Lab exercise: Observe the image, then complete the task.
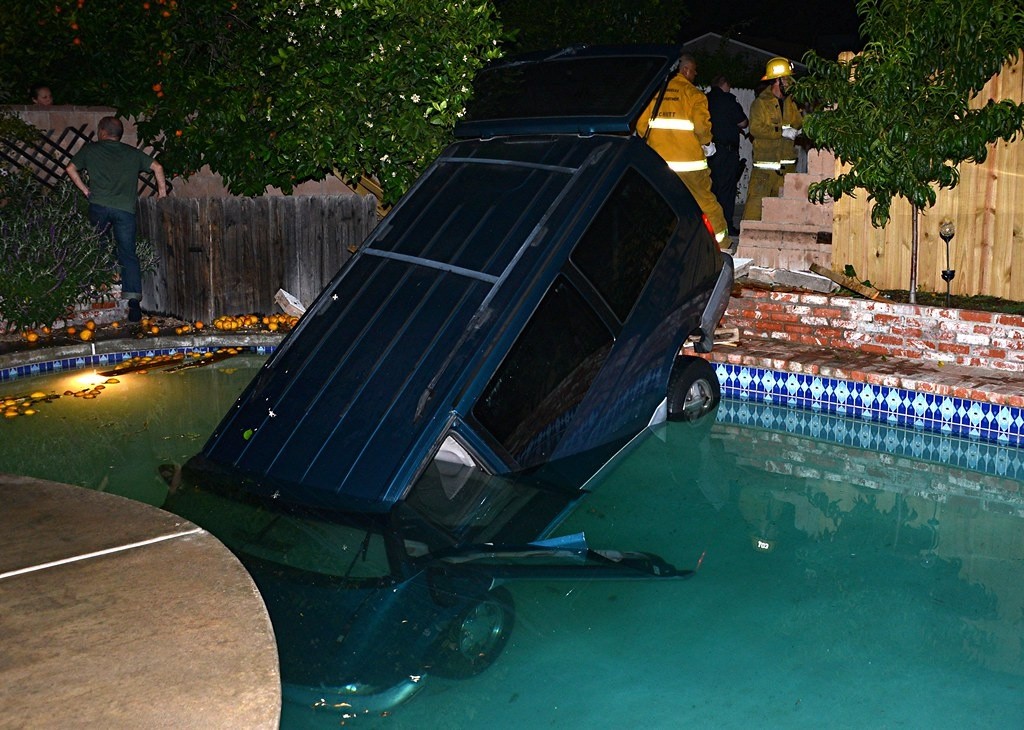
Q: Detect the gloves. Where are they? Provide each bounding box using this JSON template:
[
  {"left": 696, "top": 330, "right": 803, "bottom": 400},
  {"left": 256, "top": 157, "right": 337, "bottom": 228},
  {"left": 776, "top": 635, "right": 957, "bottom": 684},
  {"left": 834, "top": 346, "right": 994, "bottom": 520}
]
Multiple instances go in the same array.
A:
[
  {"left": 781, "top": 127, "right": 798, "bottom": 140},
  {"left": 701, "top": 142, "right": 716, "bottom": 157}
]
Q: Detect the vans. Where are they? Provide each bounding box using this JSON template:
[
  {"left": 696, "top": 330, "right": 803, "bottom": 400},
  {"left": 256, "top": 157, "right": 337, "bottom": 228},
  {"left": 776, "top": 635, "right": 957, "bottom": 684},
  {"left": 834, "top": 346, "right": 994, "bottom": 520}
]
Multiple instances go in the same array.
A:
[{"left": 157, "top": 37, "right": 736, "bottom": 717}]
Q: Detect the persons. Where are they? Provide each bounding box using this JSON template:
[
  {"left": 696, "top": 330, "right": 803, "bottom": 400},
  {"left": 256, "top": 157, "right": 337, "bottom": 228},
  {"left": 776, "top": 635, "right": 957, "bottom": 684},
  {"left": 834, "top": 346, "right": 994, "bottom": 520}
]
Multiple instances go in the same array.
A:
[
  {"left": 705, "top": 72, "right": 748, "bottom": 237},
  {"left": 741, "top": 56, "right": 803, "bottom": 219},
  {"left": 66, "top": 116, "right": 168, "bottom": 322},
  {"left": 636, "top": 56, "right": 732, "bottom": 248},
  {"left": 30, "top": 85, "right": 53, "bottom": 105}
]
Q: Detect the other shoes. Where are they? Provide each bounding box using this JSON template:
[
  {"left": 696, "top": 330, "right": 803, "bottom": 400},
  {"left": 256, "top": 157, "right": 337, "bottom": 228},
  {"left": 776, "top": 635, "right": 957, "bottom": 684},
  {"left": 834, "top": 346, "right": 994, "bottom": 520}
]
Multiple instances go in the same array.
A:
[
  {"left": 127, "top": 298, "right": 142, "bottom": 322},
  {"left": 728, "top": 226, "right": 739, "bottom": 236}
]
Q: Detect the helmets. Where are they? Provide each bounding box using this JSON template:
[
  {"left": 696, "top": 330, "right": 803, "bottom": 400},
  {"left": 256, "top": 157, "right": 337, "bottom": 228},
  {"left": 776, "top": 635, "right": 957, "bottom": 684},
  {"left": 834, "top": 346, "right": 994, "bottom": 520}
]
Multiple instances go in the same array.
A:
[{"left": 761, "top": 55, "right": 796, "bottom": 80}]
